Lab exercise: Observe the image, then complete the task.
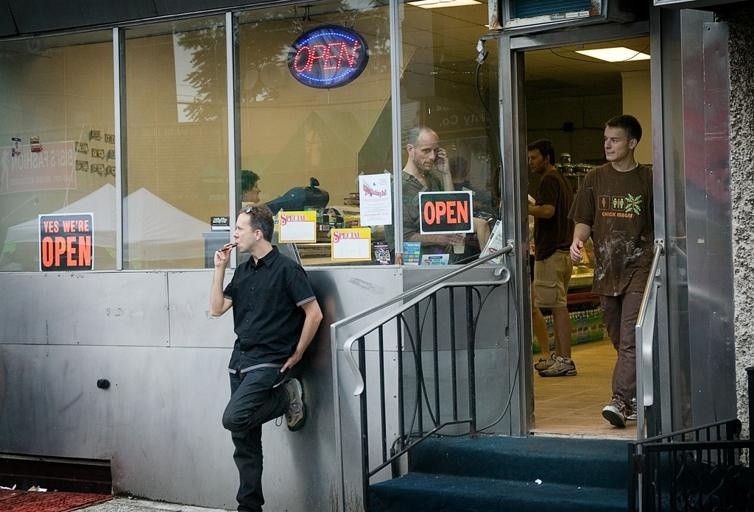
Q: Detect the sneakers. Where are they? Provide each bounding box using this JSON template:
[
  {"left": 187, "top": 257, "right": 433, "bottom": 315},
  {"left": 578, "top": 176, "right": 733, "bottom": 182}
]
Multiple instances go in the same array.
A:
[
  {"left": 283, "top": 377, "right": 306, "bottom": 432},
  {"left": 539, "top": 355, "right": 577, "bottom": 377},
  {"left": 533, "top": 351, "right": 557, "bottom": 372},
  {"left": 602, "top": 394, "right": 628, "bottom": 428},
  {"left": 626, "top": 397, "right": 637, "bottom": 420}
]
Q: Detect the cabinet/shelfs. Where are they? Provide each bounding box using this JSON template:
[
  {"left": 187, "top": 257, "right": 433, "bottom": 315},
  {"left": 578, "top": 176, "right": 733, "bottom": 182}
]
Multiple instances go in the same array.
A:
[
  {"left": 530, "top": 267, "right": 605, "bottom": 354},
  {"left": 562, "top": 172, "right": 586, "bottom": 193}
]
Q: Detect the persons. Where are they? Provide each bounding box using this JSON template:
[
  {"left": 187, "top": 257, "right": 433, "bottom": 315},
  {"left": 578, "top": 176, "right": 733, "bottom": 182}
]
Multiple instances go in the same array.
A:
[
  {"left": 240, "top": 170, "right": 262, "bottom": 204},
  {"left": 382, "top": 125, "right": 464, "bottom": 247},
  {"left": 448, "top": 156, "right": 491, "bottom": 206},
  {"left": 566, "top": 115, "right": 653, "bottom": 430},
  {"left": 210, "top": 202, "right": 324, "bottom": 511},
  {"left": 496, "top": 165, "right": 550, "bottom": 362},
  {"left": 471, "top": 216, "right": 491, "bottom": 253},
  {"left": 526, "top": 137, "right": 574, "bottom": 376}
]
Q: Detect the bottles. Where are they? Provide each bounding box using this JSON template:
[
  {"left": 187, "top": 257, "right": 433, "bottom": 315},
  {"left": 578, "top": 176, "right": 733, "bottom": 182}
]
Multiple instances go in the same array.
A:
[{"left": 533, "top": 308, "right": 606, "bottom": 354}]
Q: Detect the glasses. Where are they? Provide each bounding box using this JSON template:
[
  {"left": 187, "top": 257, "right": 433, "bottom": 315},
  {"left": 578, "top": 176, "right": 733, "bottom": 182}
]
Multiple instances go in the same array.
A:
[{"left": 240, "top": 206, "right": 267, "bottom": 230}]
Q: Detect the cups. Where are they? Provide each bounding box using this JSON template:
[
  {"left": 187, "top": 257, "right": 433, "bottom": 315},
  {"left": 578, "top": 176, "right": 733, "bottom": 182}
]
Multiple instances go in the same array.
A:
[
  {"left": 375, "top": 242, "right": 388, "bottom": 262},
  {"left": 453, "top": 233, "right": 465, "bottom": 254}
]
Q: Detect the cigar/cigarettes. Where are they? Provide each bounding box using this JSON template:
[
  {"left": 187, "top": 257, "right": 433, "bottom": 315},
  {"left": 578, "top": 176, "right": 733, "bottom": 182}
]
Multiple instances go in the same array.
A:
[{"left": 217, "top": 242, "right": 237, "bottom": 252}]
